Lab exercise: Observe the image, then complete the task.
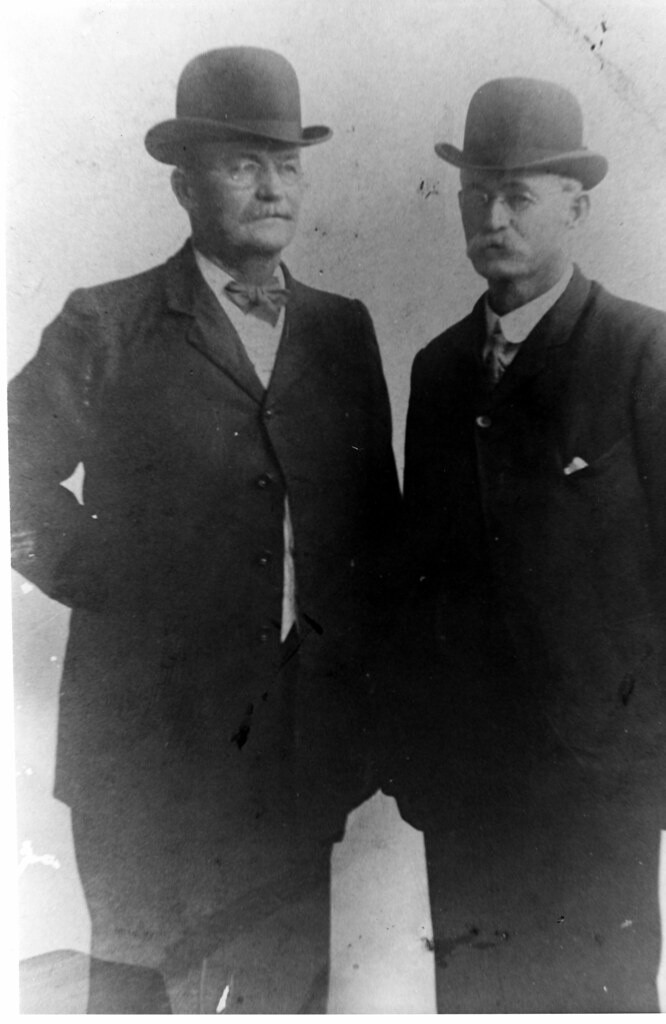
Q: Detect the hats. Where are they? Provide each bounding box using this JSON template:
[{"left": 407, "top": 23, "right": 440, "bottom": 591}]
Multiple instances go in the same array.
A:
[
  {"left": 144, "top": 45, "right": 333, "bottom": 165},
  {"left": 435, "top": 77, "right": 609, "bottom": 191}
]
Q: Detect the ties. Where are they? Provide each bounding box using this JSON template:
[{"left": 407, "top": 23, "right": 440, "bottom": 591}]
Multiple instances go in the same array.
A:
[{"left": 485, "top": 332, "right": 508, "bottom": 385}]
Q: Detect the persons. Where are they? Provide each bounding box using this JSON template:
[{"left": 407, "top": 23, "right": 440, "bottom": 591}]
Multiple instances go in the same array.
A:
[
  {"left": 7, "top": 46, "right": 400, "bottom": 1013},
  {"left": 399, "top": 74, "right": 666, "bottom": 1015}
]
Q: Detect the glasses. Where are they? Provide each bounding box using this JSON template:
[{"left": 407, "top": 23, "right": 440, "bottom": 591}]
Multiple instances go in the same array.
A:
[
  {"left": 187, "top": 154, "right": 305, "bottom": 187},
  {"left": 458, "top": 184, "right": 574, "bottom": 213}
]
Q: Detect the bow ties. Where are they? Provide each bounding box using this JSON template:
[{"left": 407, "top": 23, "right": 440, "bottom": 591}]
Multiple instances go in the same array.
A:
[{"left": 223, "top": 276, "right": 291, "bottom": 316}]
